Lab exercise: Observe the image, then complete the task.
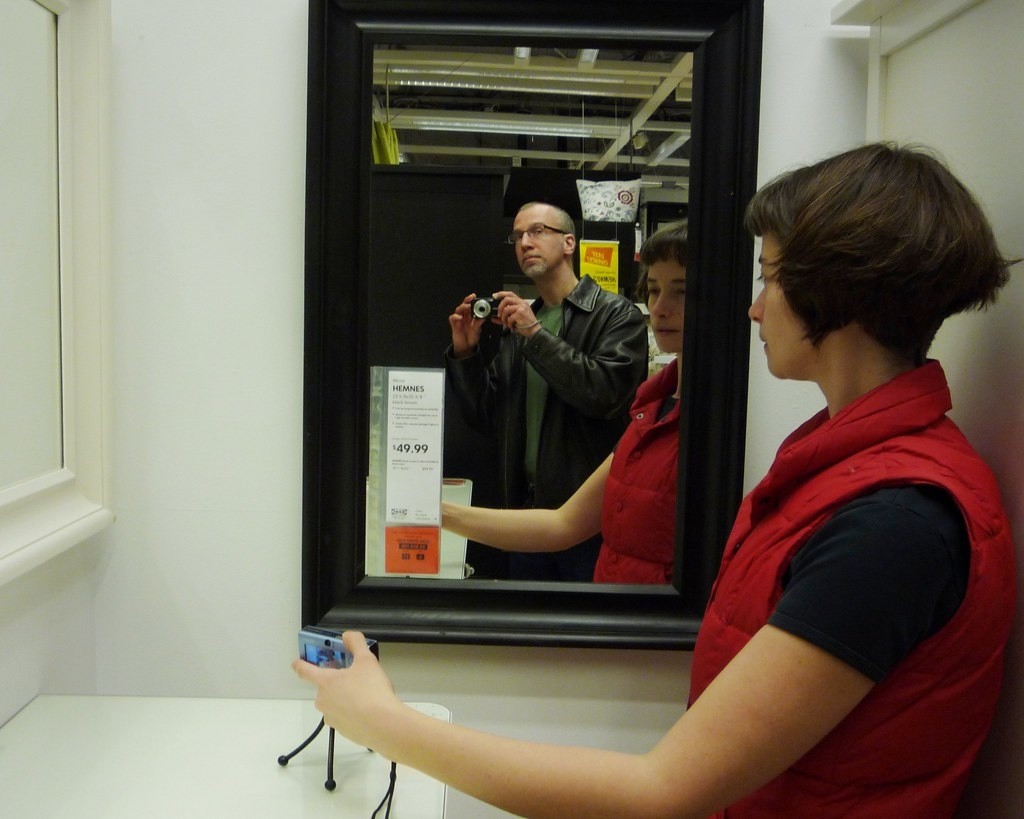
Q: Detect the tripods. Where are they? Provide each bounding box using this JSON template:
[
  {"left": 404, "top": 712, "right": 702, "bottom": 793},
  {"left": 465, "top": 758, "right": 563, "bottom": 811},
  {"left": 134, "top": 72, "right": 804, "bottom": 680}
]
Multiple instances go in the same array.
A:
[{"left": 277, "top": 712, "right": 375, "bottom": 792}]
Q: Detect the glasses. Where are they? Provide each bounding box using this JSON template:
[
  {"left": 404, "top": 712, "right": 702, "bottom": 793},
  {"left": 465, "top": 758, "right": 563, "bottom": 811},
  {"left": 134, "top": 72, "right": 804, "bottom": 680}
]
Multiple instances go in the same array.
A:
[{"left": 507, "top": 223, "right": 568, "bottom": 244}]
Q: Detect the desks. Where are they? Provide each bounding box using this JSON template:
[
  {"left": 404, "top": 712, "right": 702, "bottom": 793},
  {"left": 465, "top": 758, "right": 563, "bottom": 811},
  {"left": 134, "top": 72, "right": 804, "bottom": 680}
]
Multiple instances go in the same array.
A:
[{"left": 0, "top": 694, "right": 450, "bottom": 819}]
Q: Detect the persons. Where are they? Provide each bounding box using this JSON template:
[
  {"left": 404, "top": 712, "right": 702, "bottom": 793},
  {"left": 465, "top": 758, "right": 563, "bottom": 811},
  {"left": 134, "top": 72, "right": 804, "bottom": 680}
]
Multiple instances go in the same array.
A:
[
  {"left": 289, "top": 138, "right": 1016, "bottom": 818},
  {"left": 435, "top": 218, "right": 688, "bottom": 586},
  {"left": 439, "top": 200, "right": 652, "bottom": 581},
  {"left": 316, "top": 647, "right": 340, "bottom": 670}
]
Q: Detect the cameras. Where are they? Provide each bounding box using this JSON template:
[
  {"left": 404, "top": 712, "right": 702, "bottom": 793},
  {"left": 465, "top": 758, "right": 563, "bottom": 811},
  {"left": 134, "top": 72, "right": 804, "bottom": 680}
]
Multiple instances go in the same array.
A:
[
  {"left": 297, "top": 626, "right": 380, "bottom": 670},
  {"left": 471, "top": 297, "right": 502, "bottom": 320}
]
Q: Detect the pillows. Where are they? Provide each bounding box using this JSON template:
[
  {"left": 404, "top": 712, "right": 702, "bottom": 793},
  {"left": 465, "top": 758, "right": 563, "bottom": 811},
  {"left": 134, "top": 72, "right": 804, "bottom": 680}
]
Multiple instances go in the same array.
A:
[{"left": 574, "top": 177, "right": 642, "bottom": 224}]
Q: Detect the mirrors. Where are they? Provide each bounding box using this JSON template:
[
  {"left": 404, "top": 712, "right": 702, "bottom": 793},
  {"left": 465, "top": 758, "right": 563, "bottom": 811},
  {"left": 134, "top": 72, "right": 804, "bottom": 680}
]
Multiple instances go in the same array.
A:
[
  {"left": 1, "top": 0, "right": 120, "bottom": 588},
  {"left": 302, "top": 0, "right": 766, "bottom": 650}
]
